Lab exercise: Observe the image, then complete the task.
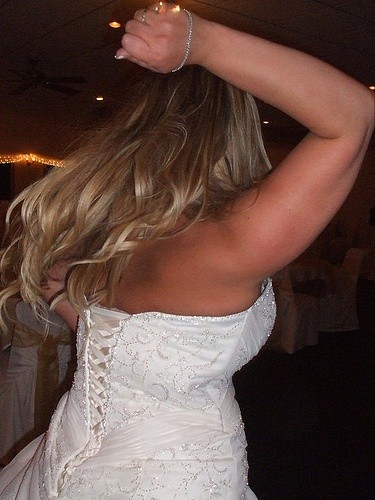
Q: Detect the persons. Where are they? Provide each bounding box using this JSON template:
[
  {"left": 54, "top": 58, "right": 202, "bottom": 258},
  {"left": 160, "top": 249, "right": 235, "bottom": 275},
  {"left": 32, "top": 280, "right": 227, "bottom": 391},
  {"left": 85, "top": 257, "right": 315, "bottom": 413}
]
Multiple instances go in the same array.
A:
[{"left": 0, "top": 0, "right": 375, "bottom": 500}]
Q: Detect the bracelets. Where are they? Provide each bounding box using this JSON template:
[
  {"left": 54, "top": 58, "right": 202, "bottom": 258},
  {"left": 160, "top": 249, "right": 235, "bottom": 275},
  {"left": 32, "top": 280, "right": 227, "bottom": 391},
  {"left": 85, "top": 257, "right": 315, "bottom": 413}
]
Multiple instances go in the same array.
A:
[{"left": 172, "top": 8, "right": 192, "bottom": 72}]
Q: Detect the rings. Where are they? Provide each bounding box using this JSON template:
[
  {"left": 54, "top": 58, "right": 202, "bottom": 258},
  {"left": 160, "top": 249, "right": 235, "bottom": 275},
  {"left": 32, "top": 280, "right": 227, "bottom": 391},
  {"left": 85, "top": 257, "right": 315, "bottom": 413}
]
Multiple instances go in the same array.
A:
[{"left": 140, "top": 9, "right": 148, "bottom": 22}]
